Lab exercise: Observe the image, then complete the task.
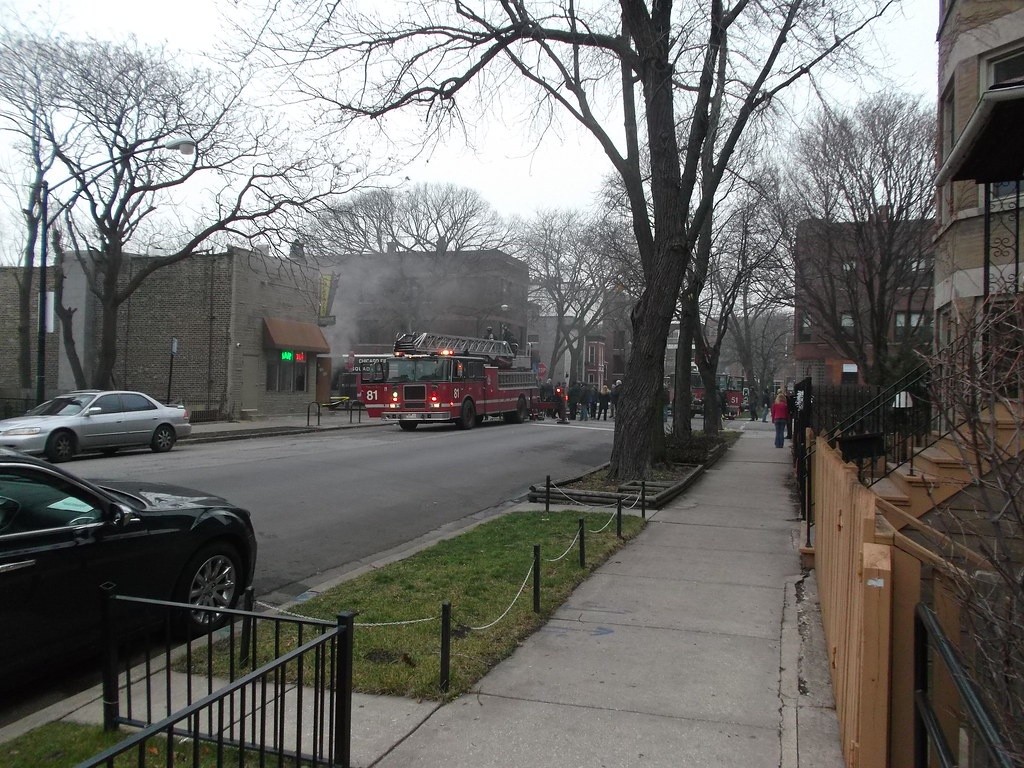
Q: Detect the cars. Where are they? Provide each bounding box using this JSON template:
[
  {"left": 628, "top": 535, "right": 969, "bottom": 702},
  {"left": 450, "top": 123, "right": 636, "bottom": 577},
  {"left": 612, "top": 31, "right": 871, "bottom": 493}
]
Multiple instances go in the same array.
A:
[
  {"left": 0, "top": 389, "right": 192, "bottom": 463},
  {"left": 0, "top": 445, "right": 257, "bottom": 678}
]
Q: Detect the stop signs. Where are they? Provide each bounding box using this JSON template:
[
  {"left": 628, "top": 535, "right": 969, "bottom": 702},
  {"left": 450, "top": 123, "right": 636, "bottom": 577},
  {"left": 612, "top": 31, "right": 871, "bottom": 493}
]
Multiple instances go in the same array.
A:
[{"left": 538, "top": 362, "right": 547, "bottom": 378}]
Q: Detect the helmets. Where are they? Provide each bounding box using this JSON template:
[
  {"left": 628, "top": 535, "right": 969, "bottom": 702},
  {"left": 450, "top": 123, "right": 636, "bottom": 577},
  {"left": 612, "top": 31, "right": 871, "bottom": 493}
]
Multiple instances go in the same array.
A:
[{"left": 487, "top": 327, "right": 493, "bottom": 331}]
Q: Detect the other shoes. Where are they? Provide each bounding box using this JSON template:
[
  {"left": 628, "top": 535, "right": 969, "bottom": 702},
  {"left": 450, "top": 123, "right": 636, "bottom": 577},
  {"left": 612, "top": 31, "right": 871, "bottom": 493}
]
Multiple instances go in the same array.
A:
[
  {"left": 755, "top": 416, "right": 758, "bottom": 420},
  {"left": 761, "top": 420, "right": 768, "bottom": 422},
  {"left": 609, "top": 415, "right": 613, "bottom": 418},
  {"left": 750, "top": 419, "right": 753, "bottom": 421},
  {"left": 579, "top": 418, "right": 583, "bottom": 421},
  {"left": 785, "top": 435, "right": 791, "bottom": 439}
]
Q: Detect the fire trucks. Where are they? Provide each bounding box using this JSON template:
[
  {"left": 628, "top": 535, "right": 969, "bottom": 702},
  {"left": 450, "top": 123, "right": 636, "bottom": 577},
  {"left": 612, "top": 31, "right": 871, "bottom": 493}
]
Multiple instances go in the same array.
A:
[
  {"left": 668, "top": 372, "right": 750, "bottom": 420},
  {"left": 346, "top": 331, "right": 570, "bottom": 430}
]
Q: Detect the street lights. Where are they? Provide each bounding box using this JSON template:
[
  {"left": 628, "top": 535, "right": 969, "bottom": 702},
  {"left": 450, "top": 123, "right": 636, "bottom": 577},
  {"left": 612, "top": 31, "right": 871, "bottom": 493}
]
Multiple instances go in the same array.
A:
[
  {"left": 476, "top": 304, "right": 508, "bottom": 337},
  {"left": 36, "top": 138, "right": 195, "bottom": 406}
]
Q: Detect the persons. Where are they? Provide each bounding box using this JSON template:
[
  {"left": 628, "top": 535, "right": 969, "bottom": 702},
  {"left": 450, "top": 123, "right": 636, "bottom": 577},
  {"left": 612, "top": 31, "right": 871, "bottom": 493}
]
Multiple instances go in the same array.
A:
[
  {"left": 748, "top": 386, "right": 759, "bottom": 422},
  {"left": 760, "top": 389, "right": 771, "bottom": 422},
  {"left": 541, "top": 378, "right": 567, "bottom": 419},
  {"left": 663, "top": 386, "right": 670, "bottom": 422},
  {"left": 714, "top": 385, "right": 729, "bottom": 421},
  {"left": 566, "top": 379, "right": 623, "bottom": 420},
  {"left": 770, "top": 388, "right": 793, "bottom": 449}
]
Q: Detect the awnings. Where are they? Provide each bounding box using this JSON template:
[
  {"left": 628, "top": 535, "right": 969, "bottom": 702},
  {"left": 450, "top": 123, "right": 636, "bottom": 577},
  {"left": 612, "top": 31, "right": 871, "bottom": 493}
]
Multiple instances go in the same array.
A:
[{"left": 262, "top": 318, "right": 330, "bottom": 355}]
